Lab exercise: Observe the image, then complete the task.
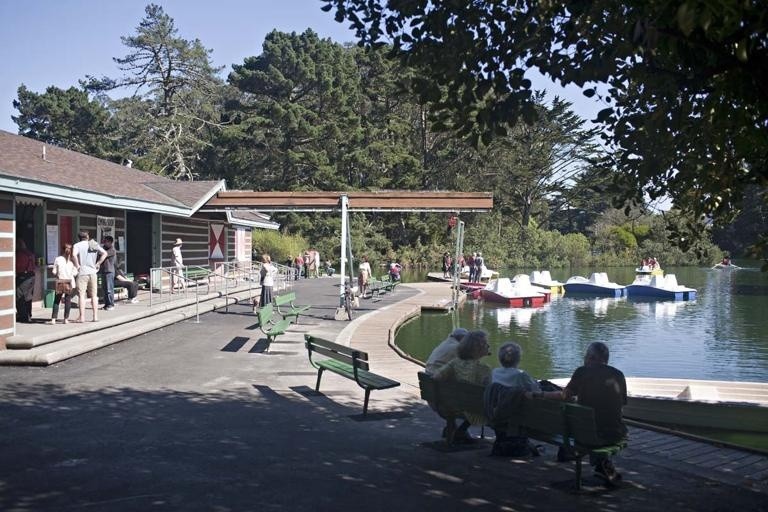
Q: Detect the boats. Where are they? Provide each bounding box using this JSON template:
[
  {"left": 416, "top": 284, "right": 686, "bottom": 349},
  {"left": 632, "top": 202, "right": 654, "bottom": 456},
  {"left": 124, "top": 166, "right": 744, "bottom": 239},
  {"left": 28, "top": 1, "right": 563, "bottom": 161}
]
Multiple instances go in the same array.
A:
[
  {"left": 635, "top": 262, "right": 664, "bottom": 275},
  {"left": 563, "top": 272, "right": 627, "bottom": 295},
  {"left": 563, "top": 292, "right": 626, "bottom": 319},
  {"left": 712, "top": 263, "right": 738, "bottom": 270},
  {"left": 480, "top": 298, "right": 543, "bottom": 328},
  {"left": 626, "top": 295, "right": 696, "bottom": 321},
  {"left": 625, "top": 274, "right": 698, "bottom": 302},
  {"left": 457, "top": 263, "right": 565, "bottom": 308}
]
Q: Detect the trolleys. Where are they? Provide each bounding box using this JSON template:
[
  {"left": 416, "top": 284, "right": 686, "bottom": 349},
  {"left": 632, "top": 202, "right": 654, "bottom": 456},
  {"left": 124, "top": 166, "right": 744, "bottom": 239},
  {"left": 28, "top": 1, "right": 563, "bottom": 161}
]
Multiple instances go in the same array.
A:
[{"left": 322, "top": 260, "right": 335, "bottom": 277}]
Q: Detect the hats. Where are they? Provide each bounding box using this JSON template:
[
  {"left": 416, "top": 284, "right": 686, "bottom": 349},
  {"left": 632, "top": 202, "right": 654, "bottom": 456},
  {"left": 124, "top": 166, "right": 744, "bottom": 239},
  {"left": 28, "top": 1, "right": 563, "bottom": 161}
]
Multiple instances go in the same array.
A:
[
  {"left": 454, "top": 328, "right": 467, "bottom": 336},
  {"left": 173, "top": 238, "right": 185, "bottom": 245}
]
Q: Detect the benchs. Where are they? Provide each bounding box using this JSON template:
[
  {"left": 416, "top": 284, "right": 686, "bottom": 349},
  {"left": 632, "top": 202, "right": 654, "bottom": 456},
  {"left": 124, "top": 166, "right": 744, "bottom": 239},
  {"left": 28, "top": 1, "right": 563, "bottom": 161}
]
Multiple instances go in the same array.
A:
[
  {"left": 96, "top": 272, "right": 148, "bottom": 299},
  {"left": 272, "top": 291, "right": 312, "bottom": 325},
  {"left": 177, "top": 265, "right": 210, "bottom": 285},
  {"left": 256, "top": 302, "right": 292, "bottom": 349},
  {"left": 366, "top": 276, "right": 389, "bottom": 297},
  {"left": 380, "top": 274, "right": 400, "bottom": 292},
  {"left": 303, "top": 333, "right": 401, "bottom": 416},
  {"left": 415, "top": 368, "right": 630, "bottom": 492}
]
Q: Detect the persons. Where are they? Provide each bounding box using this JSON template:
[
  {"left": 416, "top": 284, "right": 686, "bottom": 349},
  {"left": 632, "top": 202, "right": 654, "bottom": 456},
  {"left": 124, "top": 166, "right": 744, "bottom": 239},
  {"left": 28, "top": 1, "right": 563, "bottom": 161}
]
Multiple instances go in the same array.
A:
[
  {"left": 45, "top": 230, "right": 138, "bottom": 326},
  {"left": 426, "top": 327, "right": 471, "bottom": 442},
  {"left": 261, "top": 254, "right": 277, "bottom": 307},
  {"left": 357, "top": 256, "right": 371, "bottom": 298},
  {"left": 532, "top": 343, "right": 628, "bottom": 475},
  {"left": 722, "top": 255, "right": 731, "bottom": 265},
  {"left": 640, "top": 257, "right": 659, "bottom": 271},
  {"left": 433, "top": 331, "right": 491, "bottom": 424},
  {"left": 491, "top": 342, "right": 543, "bottom": 399},
  {"left": 443, "top": 250, "right": 484, "bottom": 283},
  {"left": 389, "top": 260, "right": 402, "bottom": 281},
  {"left": 16, "top": 238, "right": 35, "bottom": 322},
  {"left": 284, "top": 252, "right": 335, "bottom": 281},
  {"left": 171, "top": 238, "right": 186, "bottom": 291}
]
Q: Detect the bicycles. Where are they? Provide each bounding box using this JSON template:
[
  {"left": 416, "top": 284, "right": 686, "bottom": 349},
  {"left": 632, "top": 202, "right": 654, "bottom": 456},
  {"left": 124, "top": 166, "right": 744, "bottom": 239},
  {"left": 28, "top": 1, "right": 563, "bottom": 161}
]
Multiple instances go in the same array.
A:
[{"left": 332, "top": 280, "right": 357, "bottom": 321}]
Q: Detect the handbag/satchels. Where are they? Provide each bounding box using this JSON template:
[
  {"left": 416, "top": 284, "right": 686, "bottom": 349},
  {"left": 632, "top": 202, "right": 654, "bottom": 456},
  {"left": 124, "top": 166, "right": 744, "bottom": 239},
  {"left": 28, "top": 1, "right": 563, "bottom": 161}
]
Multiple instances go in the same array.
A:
[{"left": 55, "top": 279, "right": 72, "bottom": 295}]
